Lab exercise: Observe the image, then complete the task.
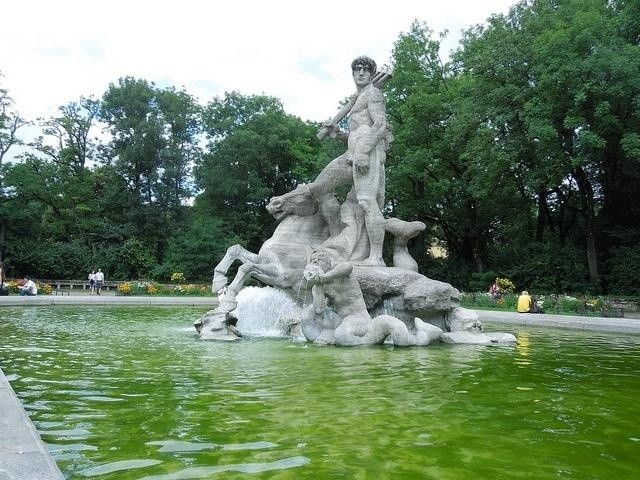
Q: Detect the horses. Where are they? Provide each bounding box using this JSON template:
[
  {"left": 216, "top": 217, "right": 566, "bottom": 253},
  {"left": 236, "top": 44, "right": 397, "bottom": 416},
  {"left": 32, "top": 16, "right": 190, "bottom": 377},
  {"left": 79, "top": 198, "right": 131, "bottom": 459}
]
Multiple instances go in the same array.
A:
[{"left": 211, "top": 182, "right": 330, "bottom": 312}]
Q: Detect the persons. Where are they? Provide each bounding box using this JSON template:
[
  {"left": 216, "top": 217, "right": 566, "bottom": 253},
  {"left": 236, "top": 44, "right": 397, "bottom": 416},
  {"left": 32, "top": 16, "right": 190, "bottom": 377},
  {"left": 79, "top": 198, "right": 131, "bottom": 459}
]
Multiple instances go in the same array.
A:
[
  {"left": 324, "top": 55, "right": 391, "bottom": 267},
  {"left": 95, "top": 268, "right": 104, "bottom": 295},
  {"left": 88, "top": 269, "right": 96, "bottom": 295},
  {"left": 16, "top": 276, "right": 37, "bottom": 296},
  {"left": 516, "top": 290, "right": 532, "bottom": 313},
  {"left": 307, "top": 248, "right": 370, "bottom": 321},
  {"left": 0, "top": 262, "right": 6, "bottom": 295},
  {"left": 489, "top": 283, "right": 500, "bottom": 294}
]
